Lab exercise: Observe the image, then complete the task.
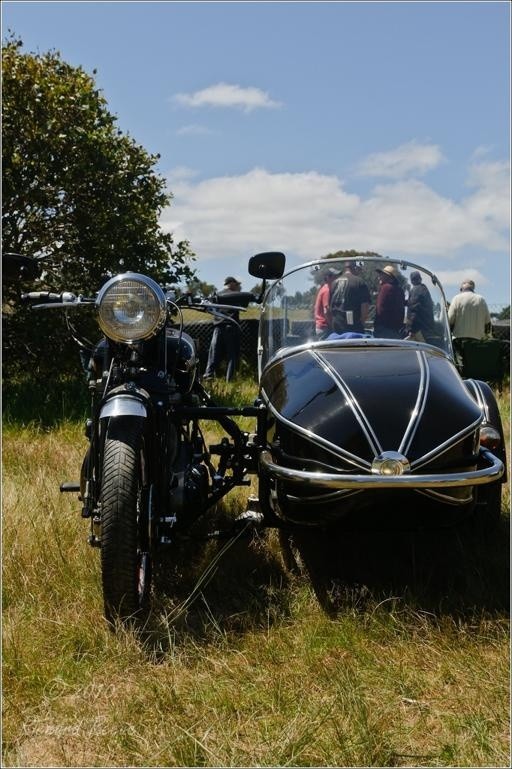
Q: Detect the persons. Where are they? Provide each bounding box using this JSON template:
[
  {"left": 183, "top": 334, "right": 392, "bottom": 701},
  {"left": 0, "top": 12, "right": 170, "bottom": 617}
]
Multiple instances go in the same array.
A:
[
  {"left": 445, "top": 278, "right": 491, "bottom": 379},
  {"left": 329, "top": 262, "right": 372, "bottom": 334},
  {"left": 405, "top": 271, "right": 435, "bottom": 342},
  {"left": 315, "top": 268, "right": 344, "bottom": 338},
  {"left": 202, "top": 276, "right": 242, "bottom": 383},
  {"left": 374, "top": 266, "right": 405, "bottom": 339}
]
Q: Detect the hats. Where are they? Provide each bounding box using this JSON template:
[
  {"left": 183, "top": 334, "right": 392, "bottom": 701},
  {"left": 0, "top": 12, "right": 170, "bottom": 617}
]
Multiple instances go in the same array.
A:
[
  {"left": 375, "top": 266, "right": 400, "bottom": 282},
  {"left": 325, "top": 267, "right": 341, "bottom": 275},
  {"left": 224, "top": 277, "right": 240, "bottom": 285}
]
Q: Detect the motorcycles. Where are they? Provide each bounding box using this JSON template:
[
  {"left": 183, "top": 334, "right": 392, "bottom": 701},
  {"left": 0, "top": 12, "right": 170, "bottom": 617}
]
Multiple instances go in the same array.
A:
[{"left": 21, "top": 251, "right": 508, "bottom": 626}]
